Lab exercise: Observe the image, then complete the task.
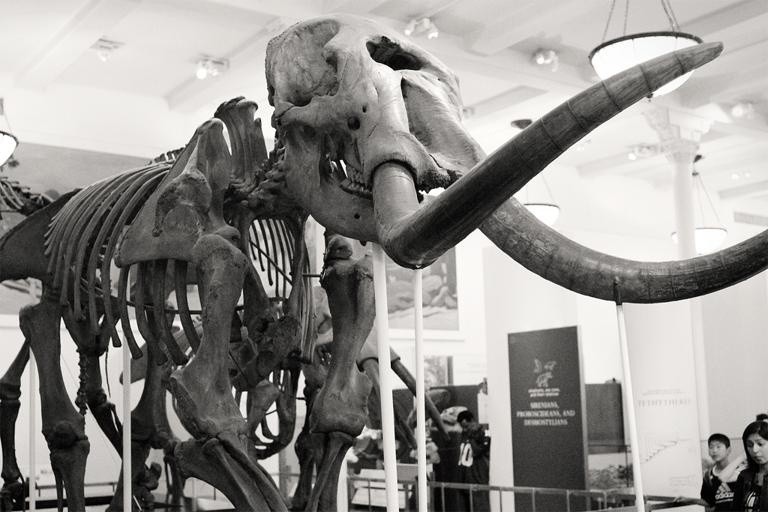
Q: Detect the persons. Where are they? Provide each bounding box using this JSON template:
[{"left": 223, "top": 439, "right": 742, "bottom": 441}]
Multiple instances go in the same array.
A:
[
  {"left": 733, "top": 421, "right": 768, "bottom": 512},
  {"left": 757, "top": 414, "right": 768, "bottom": 423},
  {"left": 455, "top": 410, "right": 489, "bottom": 512},
  {"left": 672, "top": 434, "right": 746, "bottom": 512}
]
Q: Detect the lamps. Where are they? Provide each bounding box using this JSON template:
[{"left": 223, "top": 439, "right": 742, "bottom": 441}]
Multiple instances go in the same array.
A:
[
  {"left": 534, "top": 51, "right": 560, "bottom": 71},
  {"left": 669, "top": 152, "right": 731, "bottom": 258},
  {"left": 0, "top": 97, "right": 19, "bottom": 167},
  {"left": 588, "top": 0, "right": 705, "bottom": 105},
  {"left": 191, "top": 55, "right": 231, "bottom": 79},
  {"left": 511, "top": 118, "right": 563, "bottom": 229},
  {"left": 403, "top": 21, "right": 441, "bottom": 45},
  {"left": 96, "top": 39, "right": 117, "bottom": 63}
]
